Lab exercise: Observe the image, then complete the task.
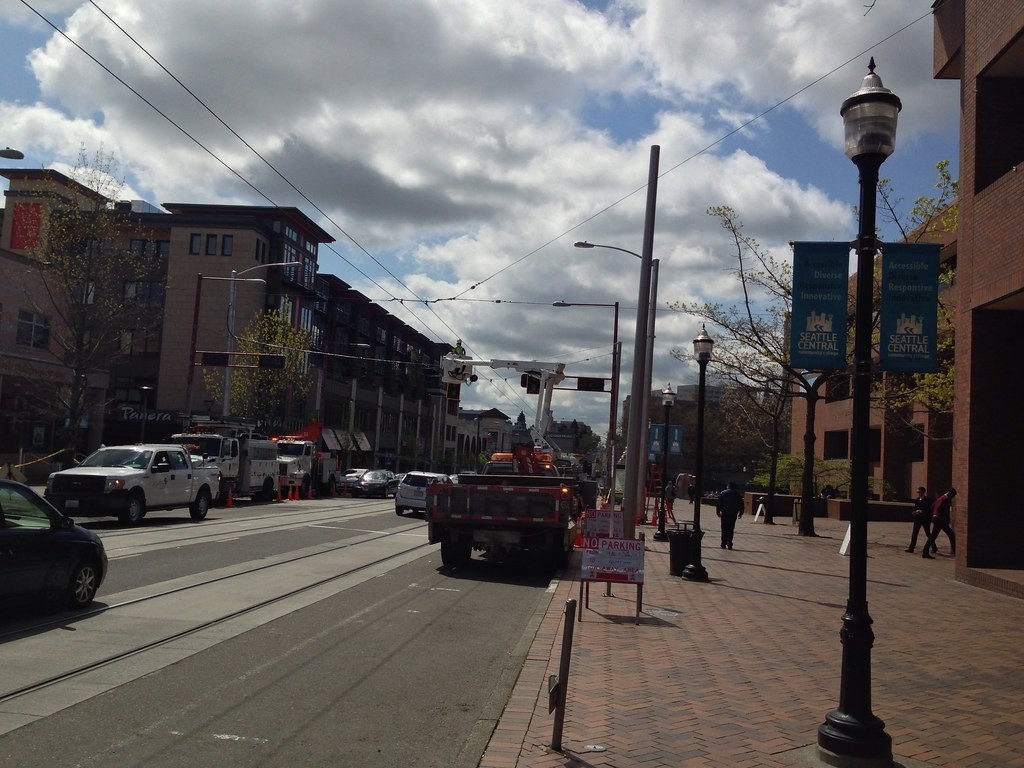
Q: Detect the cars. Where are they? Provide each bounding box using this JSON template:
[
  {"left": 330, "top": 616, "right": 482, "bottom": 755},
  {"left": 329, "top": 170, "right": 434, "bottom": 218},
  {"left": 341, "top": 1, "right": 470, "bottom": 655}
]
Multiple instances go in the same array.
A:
[
  {"left": 0, "top": 479, "right": 108, "bottom": 609},
  {"left": 394, "top": 471, "right": 452, "bottom": 515},
  {"left": 338, "top": 468, "right": 404, "bottom": 499}
]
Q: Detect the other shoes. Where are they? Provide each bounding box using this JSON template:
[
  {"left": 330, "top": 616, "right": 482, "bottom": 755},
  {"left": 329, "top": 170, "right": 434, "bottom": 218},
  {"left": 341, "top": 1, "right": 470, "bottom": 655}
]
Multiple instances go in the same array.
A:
[
  {"left": 728, "top": 546, "right": 732, "bottom": 550},
  {"left": 721, "top": 545, "right": 726, "bottom": 549},
  {"left": 905, "top": 549, "right": 914, "bottom": 553},
  {"left": 931, "top": 548, "right": 938, "bottom": 553},
  {"left": 922, "top": 553, "right": 935, "bottom": 559},
  {"left": 951, "top": 550, "right": 955, "bottom": 554}
]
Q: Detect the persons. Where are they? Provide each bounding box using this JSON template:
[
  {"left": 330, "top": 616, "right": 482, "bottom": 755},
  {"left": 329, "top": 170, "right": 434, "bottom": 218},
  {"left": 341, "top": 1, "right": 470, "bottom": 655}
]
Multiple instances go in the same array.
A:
[
  {"left": 922, "top": 488, "right": 957, "bottom": 558},
  {"left": 688, "top": 482, "right": 694, "bottom": 504},
  {"left": 716, "top": 480, "right": 745, "bottom": 549},
  {"left": 903, "top": 486, "right": 938, "bottom": 554},
  {"left": 665, "top": 481, "right": 676, "bottom": 510},
  {"left": 820, "top": 485, "right": 836, "bottom": 499}
]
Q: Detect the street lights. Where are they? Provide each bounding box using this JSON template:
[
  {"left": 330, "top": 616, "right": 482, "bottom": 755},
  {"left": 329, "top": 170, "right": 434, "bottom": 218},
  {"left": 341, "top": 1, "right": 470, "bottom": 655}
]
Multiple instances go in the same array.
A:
[
  {"left": 815, "top": 73, "right": 902, "bottom": 768},
  {"left": 576, "top": 241, "right": 660, "bottom": 527},
  {"left": 224, "top": 261, "right": 302, "bottom": 414},
  {"left": 552, "top": 302, "right": 619, "bottom": 499},
  {"left": 652, "top": 388, "right": 677, "bottom": 542},
  {"left": 304, "top": 341, "right": 371, "bottom": 372},
  {"left": 682, "top": 331, "right": 715, "bottom": 581},
  {"left": 476, "top": 421, "right": 499, "bottom": 471},
  {"left": 182, "top": 276, "right": 267, "bottom": 430}
]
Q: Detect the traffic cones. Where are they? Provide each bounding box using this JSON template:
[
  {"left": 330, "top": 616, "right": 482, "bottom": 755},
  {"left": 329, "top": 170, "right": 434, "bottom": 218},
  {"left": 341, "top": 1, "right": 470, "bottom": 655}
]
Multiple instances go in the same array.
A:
[
  {"left": 331, "top": 480, "right": 348, "bottom": 497},
  {"left": 275, "top": 487, "right": 284, "bottom": 503},
  {"left": 307, "top": 485, "right": 313, "bottom": 499},
  {"left": 225, "top": 488, "right": 235, "bottom": 508},
  {"left": 294, "top": 485, "right": 299, "bottom": 500},
  {"left": 287, "top": 485, "right": 293, "bottom": 500}
]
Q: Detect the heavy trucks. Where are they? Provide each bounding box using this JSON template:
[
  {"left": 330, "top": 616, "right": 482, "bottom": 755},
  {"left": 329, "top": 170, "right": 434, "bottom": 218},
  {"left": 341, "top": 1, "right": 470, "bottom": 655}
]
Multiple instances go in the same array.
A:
[{"left": 272, "top": 434, "right": 339, "bottom": 499}]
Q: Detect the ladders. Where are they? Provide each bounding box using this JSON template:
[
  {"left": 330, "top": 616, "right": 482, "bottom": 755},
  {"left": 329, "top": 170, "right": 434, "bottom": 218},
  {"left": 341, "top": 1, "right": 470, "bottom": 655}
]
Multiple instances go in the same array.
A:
[{"left": 645, "top": 464, "right": 676, "bottom": 522}]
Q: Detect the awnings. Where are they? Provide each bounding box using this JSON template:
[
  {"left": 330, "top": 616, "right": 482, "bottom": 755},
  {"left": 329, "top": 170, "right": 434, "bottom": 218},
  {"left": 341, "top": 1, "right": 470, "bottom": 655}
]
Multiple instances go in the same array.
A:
[
  {"left": 322, "top": 429, "right": 342, "bottom": 450},
  {"left": 335, "top": 429, "right": 357, "bottom": 451},
  {"left": 352, "top": 432, "right": 372, "bottom": 451}
]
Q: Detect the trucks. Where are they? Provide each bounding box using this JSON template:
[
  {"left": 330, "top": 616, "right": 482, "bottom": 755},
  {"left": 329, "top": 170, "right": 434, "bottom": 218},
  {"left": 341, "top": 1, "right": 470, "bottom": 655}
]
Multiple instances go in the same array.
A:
[{"left": 171, "top": 431, "right": 281, "bottom": 508}]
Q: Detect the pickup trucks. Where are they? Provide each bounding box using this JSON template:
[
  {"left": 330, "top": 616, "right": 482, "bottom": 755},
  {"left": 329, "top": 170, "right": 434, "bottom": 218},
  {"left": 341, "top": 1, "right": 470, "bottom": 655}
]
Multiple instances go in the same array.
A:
[{"left": 45, "top": 444, "right": 220, "bottom": 529}]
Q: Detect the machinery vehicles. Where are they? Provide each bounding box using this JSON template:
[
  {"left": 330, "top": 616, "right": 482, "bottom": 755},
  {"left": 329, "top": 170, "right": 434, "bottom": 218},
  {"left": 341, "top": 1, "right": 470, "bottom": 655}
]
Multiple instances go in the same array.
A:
[{"left": 425, "top": 353, "right": 583, "bottom": 578}]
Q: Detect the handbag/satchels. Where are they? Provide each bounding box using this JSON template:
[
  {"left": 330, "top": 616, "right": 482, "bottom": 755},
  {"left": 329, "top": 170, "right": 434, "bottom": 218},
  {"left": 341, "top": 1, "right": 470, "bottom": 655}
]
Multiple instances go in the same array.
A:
[{"left": 670, "top": 486, "right": 676, "bottom": 500}]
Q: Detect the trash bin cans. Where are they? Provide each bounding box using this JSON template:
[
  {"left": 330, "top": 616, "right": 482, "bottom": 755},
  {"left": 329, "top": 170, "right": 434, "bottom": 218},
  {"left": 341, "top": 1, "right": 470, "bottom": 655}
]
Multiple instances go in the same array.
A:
[
  {"left": 583, "top": 480, "right": 597, "bottom": 509},
  {"left": 669, "top": 528, "right": 706, "bottom": 576}
]
